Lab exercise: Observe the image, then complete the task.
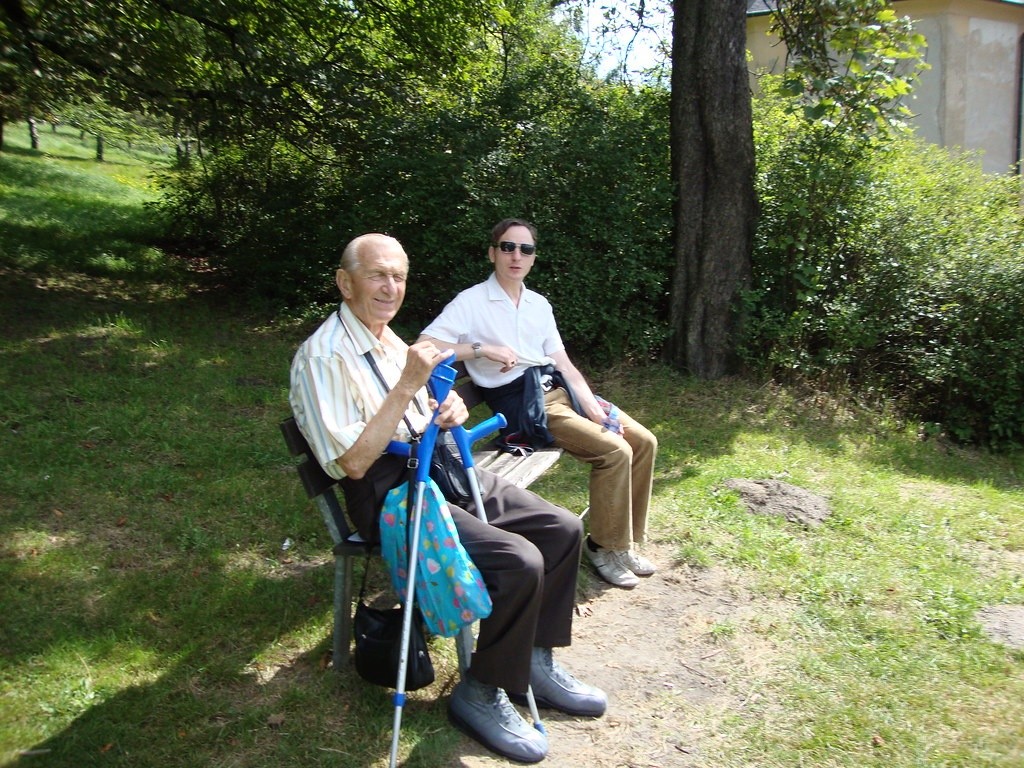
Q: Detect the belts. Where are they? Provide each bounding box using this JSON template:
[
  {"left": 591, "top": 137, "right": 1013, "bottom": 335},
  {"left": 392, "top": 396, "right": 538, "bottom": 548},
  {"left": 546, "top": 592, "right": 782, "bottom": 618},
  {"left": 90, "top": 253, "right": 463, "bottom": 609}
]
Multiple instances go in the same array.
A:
[{"left": 540, "top": 374, "right": 553, "bottom": 392}]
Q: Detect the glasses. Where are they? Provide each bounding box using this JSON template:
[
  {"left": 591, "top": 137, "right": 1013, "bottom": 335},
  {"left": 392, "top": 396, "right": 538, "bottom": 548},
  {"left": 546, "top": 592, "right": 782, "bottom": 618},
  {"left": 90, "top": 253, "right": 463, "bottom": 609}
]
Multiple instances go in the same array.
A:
[{"left": 490, "top": 240, "right": 537, "bottom": 257}]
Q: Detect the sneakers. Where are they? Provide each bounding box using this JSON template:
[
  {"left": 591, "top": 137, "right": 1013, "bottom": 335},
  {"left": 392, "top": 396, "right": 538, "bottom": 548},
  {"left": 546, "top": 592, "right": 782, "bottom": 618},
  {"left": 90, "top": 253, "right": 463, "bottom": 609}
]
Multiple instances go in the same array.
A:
[
  {"left": 447, "top": 670, "right": 548, "bottom": 762},
  {"left": 506, "top": 646, "right": 608, "bottom": 716}
]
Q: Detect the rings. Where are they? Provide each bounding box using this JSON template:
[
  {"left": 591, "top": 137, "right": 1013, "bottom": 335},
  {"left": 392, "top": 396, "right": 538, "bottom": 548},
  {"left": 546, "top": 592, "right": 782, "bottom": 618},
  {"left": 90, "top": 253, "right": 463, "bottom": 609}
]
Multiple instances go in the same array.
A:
[{"left": 511, "top": 361, "right": 514, "bottom": 364}]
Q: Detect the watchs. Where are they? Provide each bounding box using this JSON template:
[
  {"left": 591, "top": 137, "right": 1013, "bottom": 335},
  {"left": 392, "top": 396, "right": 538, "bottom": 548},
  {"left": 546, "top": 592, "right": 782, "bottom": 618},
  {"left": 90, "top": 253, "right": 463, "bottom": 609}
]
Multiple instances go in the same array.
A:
[{"left": 472, "top": 342, "right": 482, "bottom": 359}]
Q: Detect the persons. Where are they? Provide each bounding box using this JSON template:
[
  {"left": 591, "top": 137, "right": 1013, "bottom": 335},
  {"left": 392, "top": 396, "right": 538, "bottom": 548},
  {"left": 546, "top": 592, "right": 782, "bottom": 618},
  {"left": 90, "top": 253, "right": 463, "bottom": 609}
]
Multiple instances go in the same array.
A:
[
  {"left": 289, "top": 232, "right": 608, "bottom": 764},
  {"left": 411, "top": 218, "right": 659, "bottom": 589}
]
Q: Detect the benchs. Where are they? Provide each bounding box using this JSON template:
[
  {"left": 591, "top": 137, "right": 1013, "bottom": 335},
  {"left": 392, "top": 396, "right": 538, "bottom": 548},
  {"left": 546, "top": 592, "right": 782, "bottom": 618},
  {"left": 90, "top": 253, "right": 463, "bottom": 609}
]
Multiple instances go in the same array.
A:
[{"left": 280, "top": 360, "right": 566, "bottom": 686}]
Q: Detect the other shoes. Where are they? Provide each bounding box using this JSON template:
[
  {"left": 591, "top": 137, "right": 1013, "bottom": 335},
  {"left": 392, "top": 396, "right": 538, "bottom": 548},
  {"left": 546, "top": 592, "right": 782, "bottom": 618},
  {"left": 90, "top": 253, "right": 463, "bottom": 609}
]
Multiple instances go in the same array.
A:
[
  {"left": 582, "top": 539, "right": 640, "bottom": 587},
  {"left": 612, "top": 548, "right": 657, "bottom": 575}
]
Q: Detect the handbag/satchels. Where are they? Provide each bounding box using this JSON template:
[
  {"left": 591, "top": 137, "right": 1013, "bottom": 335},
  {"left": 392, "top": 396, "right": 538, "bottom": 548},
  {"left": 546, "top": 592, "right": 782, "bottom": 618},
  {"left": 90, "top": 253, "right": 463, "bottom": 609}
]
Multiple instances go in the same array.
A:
[
  {"left": 379, "top": 475, "right": 493, "bottom": 638},
  {"left": 420, "top": 431, "right": 472, "bottom": 508},
  {"left": 353, "top": 602, "right": 435, "bottom": 692}
]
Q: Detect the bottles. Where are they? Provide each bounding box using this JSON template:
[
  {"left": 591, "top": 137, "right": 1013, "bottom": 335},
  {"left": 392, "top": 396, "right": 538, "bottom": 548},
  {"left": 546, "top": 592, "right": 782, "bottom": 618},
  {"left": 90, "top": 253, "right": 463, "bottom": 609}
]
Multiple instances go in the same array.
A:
[{"left": 602, "top": 411, "right": 621, "bottom": 434}]
